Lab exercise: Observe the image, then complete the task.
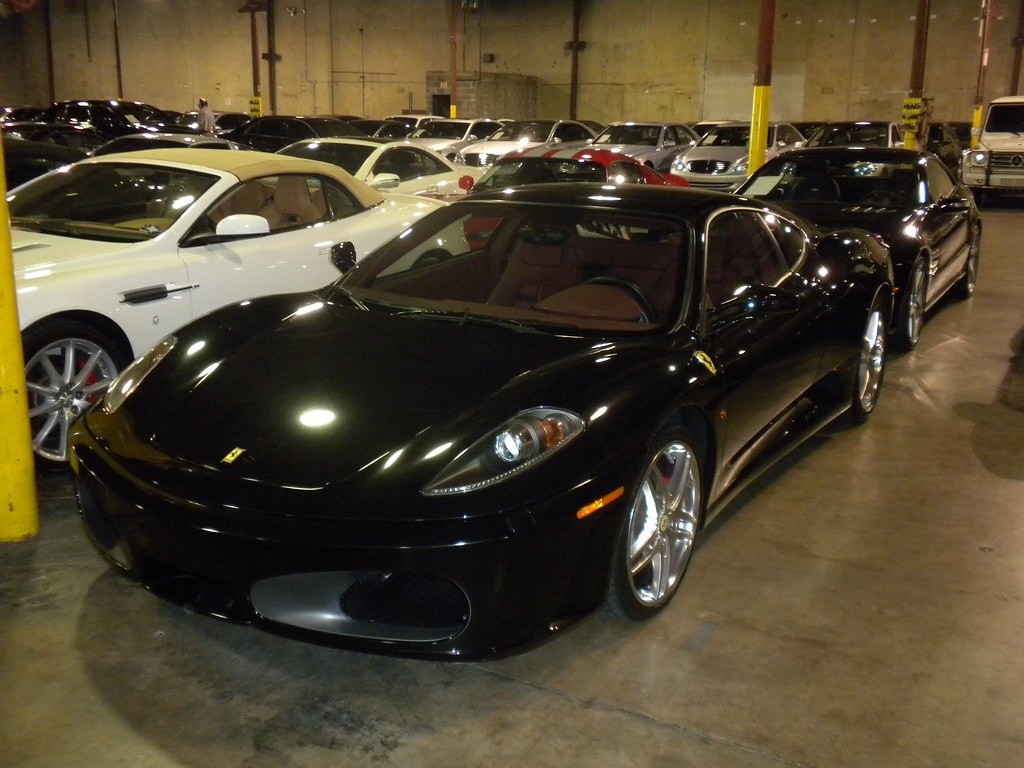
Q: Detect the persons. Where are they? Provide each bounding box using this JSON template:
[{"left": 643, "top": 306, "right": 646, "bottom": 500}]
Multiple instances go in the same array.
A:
[{"left": 197, "top": 97, "right": 215, "bottom": 130}]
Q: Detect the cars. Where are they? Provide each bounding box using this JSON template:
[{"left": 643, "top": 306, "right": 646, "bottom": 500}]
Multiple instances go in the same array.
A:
[
  {"left": 1, "top": 94, "right": 946, "bottom": 478},
  {"left": 734, "top": 143, "right": 981, "bottom": 353},
  {"left": 64, "top": 186, "right": 894, "bottom": 661},
  {"left": 956, "top": 96, "right": 1024, "bottom": 202}
]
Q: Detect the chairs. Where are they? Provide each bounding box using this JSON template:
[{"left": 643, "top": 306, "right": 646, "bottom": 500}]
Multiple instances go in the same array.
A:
[
  {"left": 879, "top": 168, "right": 921, "bottom": 206},
  {"left": 164, "top": 178, "right": 227, "bottom": 225},
  {"left": 785, "top": 160, "right": 842, "bottom": 204},
  {"left": 487, "top": 231, "right": 585, "bottom": 311},
  {"left": 612, "top": 241, "right": 682, "bottom": 322},
  {"left": 645, "top": 128, "right": 668, "bottom": 147},
  {"left": 257, "top": 176, "right": 320, "bottom": 232}
]
